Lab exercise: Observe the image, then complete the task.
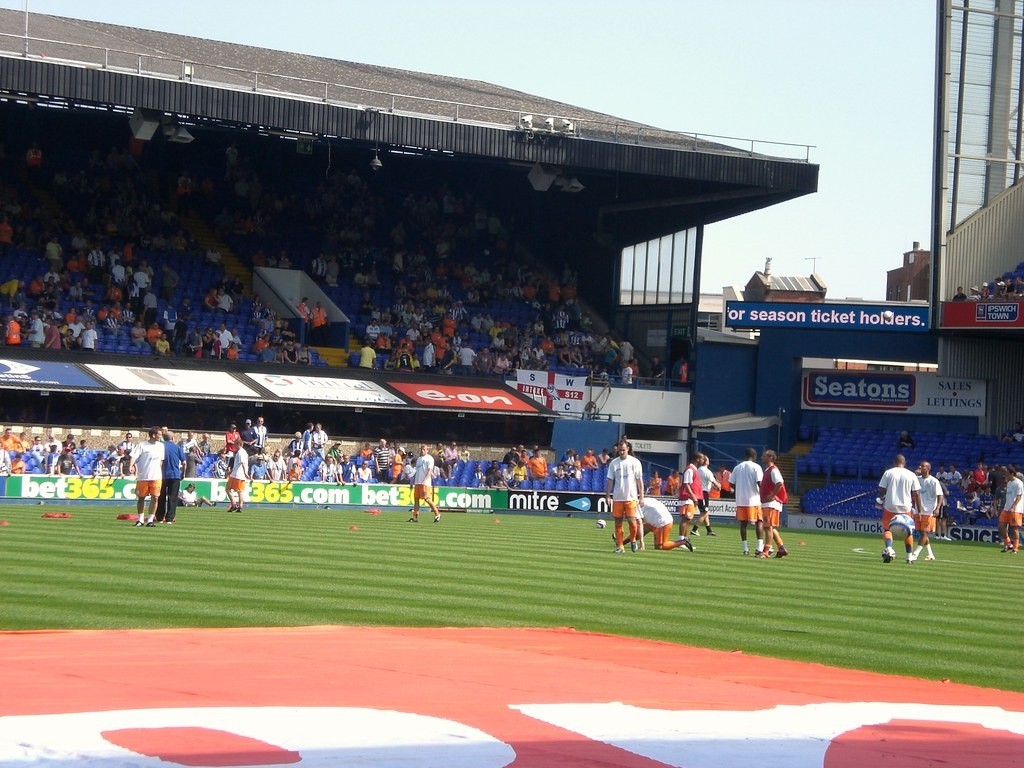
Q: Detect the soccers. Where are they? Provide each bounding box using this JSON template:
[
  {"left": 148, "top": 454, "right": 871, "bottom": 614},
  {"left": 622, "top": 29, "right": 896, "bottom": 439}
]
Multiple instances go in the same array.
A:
[{"left": 882, "top": 548, "right": 896, "bottom": 560}]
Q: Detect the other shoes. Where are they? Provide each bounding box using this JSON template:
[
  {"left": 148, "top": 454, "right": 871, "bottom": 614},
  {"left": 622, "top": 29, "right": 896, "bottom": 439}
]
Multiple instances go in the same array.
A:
[
  {"left": 407, "top": 518, "right": 419, "bottom": 523},
  {"left": 229, "top": 506, "right": 238, "bottom": 513},
  {"left": 882, "top": 552, "right": 935, "bottom": 564},
  {"left": 133, "top": 521, "right": 143, "bottom": 527},
  {"left": 1009, "top": 550, "right": 1018, "bottom": 554},
  {"left": 146, "top": 522, "right": 156, "bottom": 528},
  {"left": 1000, "top": 544, "right": 1013, "bottom": 552},
  {"left": 707, "top": 531, "right": 716, "bottom": 536},
  {"left": 690, "top": 530, "right": 700, "bottom": 536},
  {"left": 235, "top": 508, "right": 242, "bottom": 513},
  {"left": 434, "top": 513, "right": 440, "bottom": 521},
  {"left": 935, "top": 535, "right": 951, "bottom": 541},
  {"left": 684, "top": 538, "right": 693, "bottom": 551},
  {"left": 632, "top": 540, "right": 639, "bottom": 553},
  {"left": 611, "top": 548, "right": 625, "bottom": 554}
]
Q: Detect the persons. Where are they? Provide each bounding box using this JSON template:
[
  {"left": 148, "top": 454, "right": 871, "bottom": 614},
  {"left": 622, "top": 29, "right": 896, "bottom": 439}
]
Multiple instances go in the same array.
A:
[
  {"left": 648, "top": 472, "right": 662, "bottom": 495},
  {"left": 952, "top": 277, "right": 1024, "bottom": 301},
  {"left": 936, "top": 461, "right": 1024, "bottom": 546},
  {"left": 728, "top": 448, "right": 773, "bottom": 555},
  {"left": 0, "top": 131, "right": 690, "bottom": 386},
  {"left": 664, "top": 470, "right": 680, "bottom": 495},
  {"left": 755, "top": 450, "right": 789, "bottom": 558},
  {"left": 129, "top": 427, "right": 187, "bottom": 527},
  {"left": 897, "top": 431, "right": 914, "bottom": 449},
  {"left": 1003, "top": 422, "right": 1024, "bottom": 441},
  {"left": 690, "top": 456, "right": 720, "bottom": 535},
  {"left": 0, "top": 415, "right": 632, "bottom": 487},
  {"left": 717, "top": 464, "right": 734, "bottom": 498},
  {"left": 879, "top": 455, "right": 943, "bottom": 560},
  {"left": 612, "top": 498, "right": 693, "bottom": 552},
  {"left": 606, "top": 442, "right": 644, "bottom": 553},
  {"left": 678, "top": 453, "right": 704, "bottom": 549},
  {"left": 409, "top": 445, "right": 441, "bottom": 522},
  {"left": 226, "top": 440, "right": 249, "bottom": 512},
  {"left": 165, "top": 484, "right": 218, "bottom": 507},
  {"left": 1000, "top": 467, "right": 1024, "bottom": 554}
]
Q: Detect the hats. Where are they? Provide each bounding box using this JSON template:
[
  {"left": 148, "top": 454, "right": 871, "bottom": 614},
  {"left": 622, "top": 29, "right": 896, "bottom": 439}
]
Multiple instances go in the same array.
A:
[
  {"left": 400, "top": 447, "right": 406, "bottom": 455},
  {"left": 64, "top": 446, "right": 71, "bottom": 451},
  {"left": 245, "top": 419, "right": 252, "bottom": 423}
]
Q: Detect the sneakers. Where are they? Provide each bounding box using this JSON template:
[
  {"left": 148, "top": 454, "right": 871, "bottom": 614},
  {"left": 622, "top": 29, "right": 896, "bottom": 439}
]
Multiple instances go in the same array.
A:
[{"left": 743, "top": 546, "right": 788, "bottom": 559}]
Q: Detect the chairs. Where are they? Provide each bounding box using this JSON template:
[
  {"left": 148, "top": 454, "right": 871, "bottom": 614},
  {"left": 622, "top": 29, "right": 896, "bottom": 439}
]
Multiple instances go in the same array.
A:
[
  {"left": 0, "top": 223, "right": 620, "bottom": 380},
  {"left": 986, "top": 262, "right": 1024, "bottom": 292},
  {"left": 10, "top": 446, "right": 610, "bottom": 492},
  {"left": 797, "top": 426, "right": 1024, "bottom": 526}
]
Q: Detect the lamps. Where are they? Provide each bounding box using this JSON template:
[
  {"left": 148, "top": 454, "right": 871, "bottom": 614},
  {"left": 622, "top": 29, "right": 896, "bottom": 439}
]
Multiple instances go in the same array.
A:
[
  {"left": 167, "top": 127, "right": 195, "bottom": 144},
  {"left": 370, "top": 156, "right": 382, "bottom": 171},
  {"left": 561, "top": 179, "right": 584, "bottom": 192}
]
